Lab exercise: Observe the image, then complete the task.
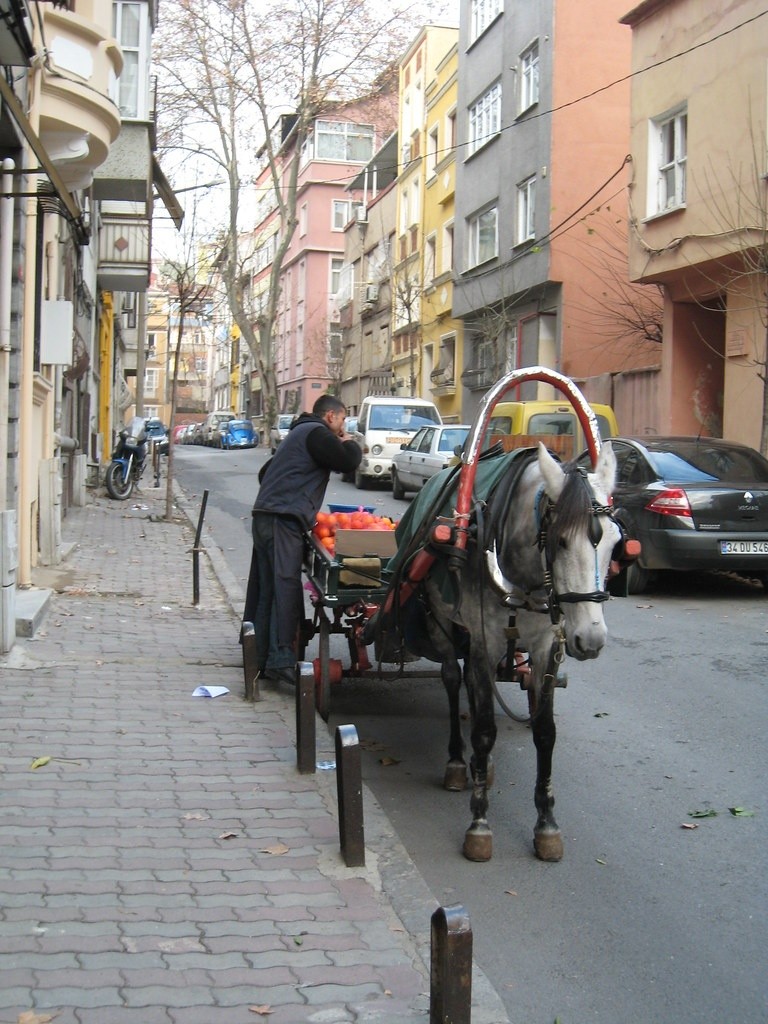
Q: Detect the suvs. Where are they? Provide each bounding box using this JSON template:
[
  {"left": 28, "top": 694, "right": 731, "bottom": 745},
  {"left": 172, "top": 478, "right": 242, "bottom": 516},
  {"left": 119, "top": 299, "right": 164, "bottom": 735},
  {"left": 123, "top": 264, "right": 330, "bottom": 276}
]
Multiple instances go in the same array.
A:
[
  {"left": 143, "top": 417, "right": 170, "bottom": 457},
  {"left": 270, "top": 413, "right": 299, "bottom": 455}
]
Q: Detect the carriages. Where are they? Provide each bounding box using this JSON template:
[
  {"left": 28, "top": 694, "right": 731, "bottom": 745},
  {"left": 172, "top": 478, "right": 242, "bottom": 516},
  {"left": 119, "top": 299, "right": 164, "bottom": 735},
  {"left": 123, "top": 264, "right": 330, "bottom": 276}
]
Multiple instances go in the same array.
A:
[{"left": 303, "top": 365, "right": 640, "bottom": 865}]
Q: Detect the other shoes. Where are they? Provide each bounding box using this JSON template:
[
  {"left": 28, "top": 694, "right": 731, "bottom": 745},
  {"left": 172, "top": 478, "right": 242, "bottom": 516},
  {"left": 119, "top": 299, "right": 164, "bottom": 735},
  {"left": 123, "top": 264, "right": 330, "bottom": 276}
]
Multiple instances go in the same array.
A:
[{"left": 264, "top": 667, "right": 297, "bottom": 686}]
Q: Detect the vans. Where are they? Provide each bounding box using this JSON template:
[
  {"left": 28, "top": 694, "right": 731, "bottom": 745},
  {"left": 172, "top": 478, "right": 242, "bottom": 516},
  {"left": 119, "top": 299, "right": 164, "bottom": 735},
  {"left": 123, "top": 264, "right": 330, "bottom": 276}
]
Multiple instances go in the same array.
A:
[
  {"left": 450, "top": 401, "right": 617, "bottom": 468},
  {"left": 352, "top": 395, "right": 445, "bottom": 490}
]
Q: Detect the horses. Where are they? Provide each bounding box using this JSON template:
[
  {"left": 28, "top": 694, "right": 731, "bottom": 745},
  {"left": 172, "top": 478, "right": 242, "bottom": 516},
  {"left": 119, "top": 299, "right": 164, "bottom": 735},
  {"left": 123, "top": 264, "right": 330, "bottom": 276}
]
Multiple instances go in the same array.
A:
[{"left": 402, "top": 442, "right": 622, "bottom": 863}]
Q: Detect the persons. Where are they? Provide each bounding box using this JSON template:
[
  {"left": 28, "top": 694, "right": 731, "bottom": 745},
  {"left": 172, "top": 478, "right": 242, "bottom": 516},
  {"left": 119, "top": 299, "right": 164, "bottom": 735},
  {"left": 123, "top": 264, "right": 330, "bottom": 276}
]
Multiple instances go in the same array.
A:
[{"left": 238, "top": 394, "right": 364, "bottom": 685}]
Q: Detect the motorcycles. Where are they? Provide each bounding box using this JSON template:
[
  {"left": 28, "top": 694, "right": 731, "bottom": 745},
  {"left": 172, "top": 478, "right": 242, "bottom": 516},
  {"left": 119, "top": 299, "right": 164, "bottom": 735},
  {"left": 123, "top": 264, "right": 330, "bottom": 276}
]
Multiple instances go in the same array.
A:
[{"left": 106, "top": 417, "right": 160, "bottom": 500}]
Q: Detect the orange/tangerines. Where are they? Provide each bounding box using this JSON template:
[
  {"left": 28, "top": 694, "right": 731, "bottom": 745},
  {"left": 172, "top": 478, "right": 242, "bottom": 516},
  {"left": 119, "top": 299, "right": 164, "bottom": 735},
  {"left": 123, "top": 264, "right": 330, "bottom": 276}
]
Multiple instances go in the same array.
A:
[{"left": 314, "top": 512, "right": 398, "bottom": 558}]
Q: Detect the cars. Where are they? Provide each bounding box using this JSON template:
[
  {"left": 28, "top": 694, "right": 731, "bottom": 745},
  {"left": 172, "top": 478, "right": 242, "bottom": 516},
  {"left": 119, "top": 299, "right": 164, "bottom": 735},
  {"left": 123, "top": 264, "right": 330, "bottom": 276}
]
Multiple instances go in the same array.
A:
[
  {"left": 570, "top": 436, "right": 768, "bottom": 595},
  {"left": 173, "top": 411, "right": 258, "bottom": 451},
  {"left": 391, "top": 423, "right": 474, "bottom": 500}
]
